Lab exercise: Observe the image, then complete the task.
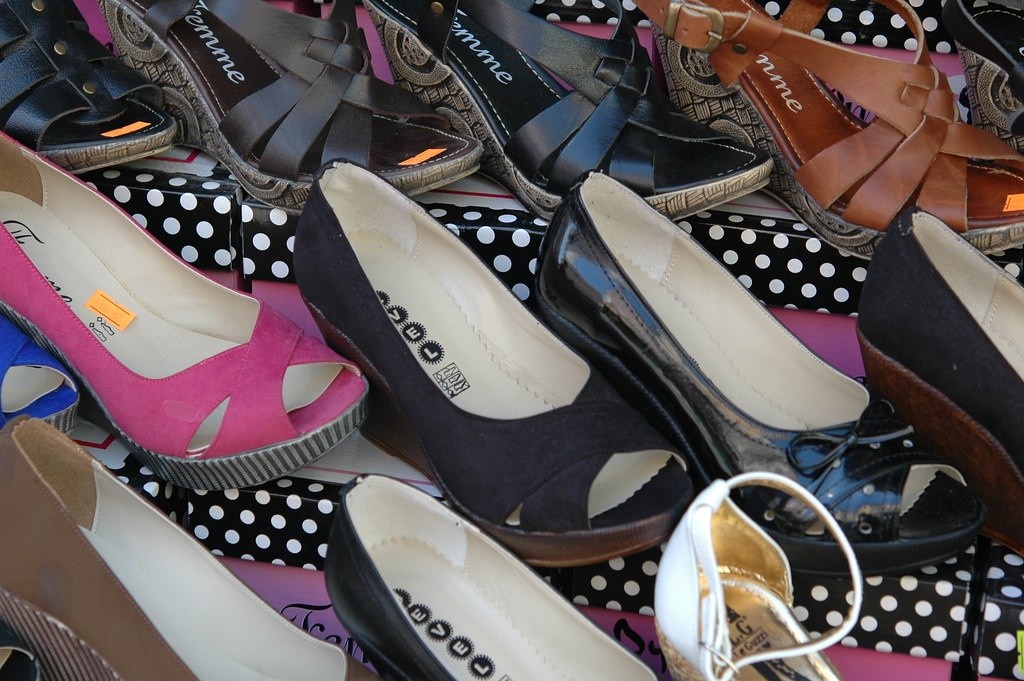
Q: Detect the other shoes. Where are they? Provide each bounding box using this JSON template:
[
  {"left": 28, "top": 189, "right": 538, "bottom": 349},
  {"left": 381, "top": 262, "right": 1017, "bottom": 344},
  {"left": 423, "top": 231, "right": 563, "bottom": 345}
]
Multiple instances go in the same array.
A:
[{"left": 0, "top": 1, "right": 1024, "bottom": 680}]
